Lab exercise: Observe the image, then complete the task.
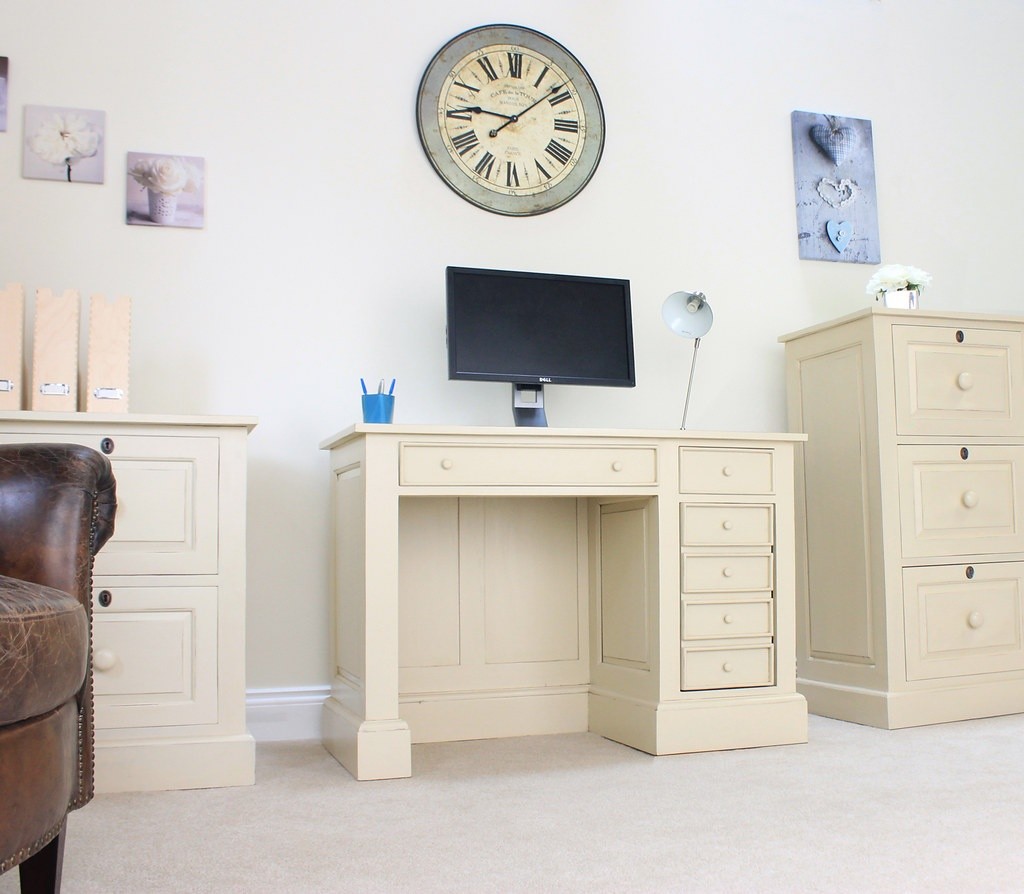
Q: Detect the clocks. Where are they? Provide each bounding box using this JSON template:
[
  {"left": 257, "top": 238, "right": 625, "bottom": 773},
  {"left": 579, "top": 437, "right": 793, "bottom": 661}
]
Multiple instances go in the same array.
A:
[{"left": 415, "top": 23, "right": 605, "bottom": 216}]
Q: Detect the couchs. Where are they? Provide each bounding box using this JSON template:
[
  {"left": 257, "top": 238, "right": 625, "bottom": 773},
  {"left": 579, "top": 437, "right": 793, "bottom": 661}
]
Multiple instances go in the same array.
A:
[{"left": 0, "top": 442, "right": 117, "bottom": 894}]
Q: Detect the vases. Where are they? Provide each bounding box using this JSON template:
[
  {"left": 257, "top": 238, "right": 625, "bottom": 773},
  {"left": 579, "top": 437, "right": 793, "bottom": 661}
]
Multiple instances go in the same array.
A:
[{"left": 878, "top": 290, "right": 920, "bottom": 309}]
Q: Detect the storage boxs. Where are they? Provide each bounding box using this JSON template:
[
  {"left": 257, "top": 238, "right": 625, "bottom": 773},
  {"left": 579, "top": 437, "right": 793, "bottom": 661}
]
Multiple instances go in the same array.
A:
[{"left": 0, "top": 282, "right": 133, "bottom": 413}]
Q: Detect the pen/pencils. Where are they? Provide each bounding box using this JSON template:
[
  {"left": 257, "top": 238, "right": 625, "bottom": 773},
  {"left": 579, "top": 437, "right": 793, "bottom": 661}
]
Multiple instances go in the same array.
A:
[{"left": 359, "top": 378, "right": 396, "bottom": 395}]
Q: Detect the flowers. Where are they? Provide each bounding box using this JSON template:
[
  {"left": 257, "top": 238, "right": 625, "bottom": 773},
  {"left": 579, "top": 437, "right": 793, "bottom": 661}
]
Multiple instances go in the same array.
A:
[{"left": 867, "top": 263, "right": 933, "bottom": 301}]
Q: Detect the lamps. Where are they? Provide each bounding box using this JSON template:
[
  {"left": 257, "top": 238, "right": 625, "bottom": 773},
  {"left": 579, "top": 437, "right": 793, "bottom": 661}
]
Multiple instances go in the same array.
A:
[{"left": 662, "top": 291, "right": 714, "bottom": 430}]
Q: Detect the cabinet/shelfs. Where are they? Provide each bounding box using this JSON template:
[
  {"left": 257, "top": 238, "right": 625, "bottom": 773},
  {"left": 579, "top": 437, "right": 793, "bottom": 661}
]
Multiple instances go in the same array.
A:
[{"left": 778, "top": 306, "right": 1024, "bottom": 729}]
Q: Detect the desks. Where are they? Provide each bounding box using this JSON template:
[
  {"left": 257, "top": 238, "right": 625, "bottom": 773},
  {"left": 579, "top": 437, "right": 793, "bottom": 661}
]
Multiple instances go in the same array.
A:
[
  {"left": 0, "top": 410, "right": 260, "bottom": 792},
  {"left": 318, "top": 422, "right": 810, "bottom": 782}
]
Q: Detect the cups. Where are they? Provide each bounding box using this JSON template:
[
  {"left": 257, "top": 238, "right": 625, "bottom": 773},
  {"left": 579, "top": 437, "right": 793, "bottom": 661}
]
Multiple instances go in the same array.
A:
[{"left": 363, "top": 393, "right": 395, "bottom": 423}]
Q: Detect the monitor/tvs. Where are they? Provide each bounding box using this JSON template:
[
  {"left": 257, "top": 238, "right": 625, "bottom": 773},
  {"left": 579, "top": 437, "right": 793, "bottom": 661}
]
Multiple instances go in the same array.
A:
[{"left": 446, "top": 266, "right": 636, "bottom": 427}]
